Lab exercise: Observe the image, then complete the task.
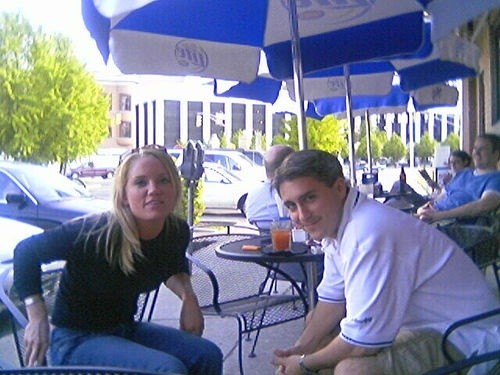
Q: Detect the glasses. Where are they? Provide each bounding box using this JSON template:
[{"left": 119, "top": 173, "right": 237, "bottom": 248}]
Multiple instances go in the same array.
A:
[{"left": 118, "top": 144, "right": 169, "bottom": 168}]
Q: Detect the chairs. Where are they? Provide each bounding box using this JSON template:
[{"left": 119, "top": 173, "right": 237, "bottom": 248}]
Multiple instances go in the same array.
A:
[
  {"left": 434, "top": 214, "right": 500, "bottom": 291},
  {"left": 0, "top": 260, "right": 161, "bottom": 375},
  {"left": 420, "top": 307, "right": 500, "bottom": 375},
  {"left": 183, "top": 217, "right": 325, "bottom": 375}
]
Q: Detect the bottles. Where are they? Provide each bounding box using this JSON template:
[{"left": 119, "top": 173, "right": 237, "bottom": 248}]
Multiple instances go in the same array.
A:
[{"left": 400, "top": 167, "right": 406, "bottom": 193}]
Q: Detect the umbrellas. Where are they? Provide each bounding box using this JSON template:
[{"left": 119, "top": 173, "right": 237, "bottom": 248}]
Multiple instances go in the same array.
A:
[
  {"left": 81, "top": 0, "right": 500, "bottom": 152},
  {"left": 214, "top": 33, "right": 482, "bottom": 190},
  {"left": 303, "top": 80, "right": 460, "bottom": 172}
]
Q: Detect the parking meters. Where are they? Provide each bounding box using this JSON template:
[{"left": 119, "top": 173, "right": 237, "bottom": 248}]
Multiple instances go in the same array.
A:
[{"left": 180, "top": 142, "right": 204, "bottom": 276}]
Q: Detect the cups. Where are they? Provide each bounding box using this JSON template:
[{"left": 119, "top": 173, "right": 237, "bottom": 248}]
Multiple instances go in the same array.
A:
[
  {"left": 292, "top": 229, "right": 308, "bottom": 243},
  {"left": 271, "top": 220, "right": 292, "bottom": 251},
  {"left": 360, "top": 184, "right": 374, "bottom": 199}
]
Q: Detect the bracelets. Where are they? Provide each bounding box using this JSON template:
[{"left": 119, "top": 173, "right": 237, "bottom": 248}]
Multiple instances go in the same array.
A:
[
  {"left": 24, "top": 294, "right": 45, "bottom": 306},
  {"left": 299, "top": 353, "right": 315, "bottom": 373}
]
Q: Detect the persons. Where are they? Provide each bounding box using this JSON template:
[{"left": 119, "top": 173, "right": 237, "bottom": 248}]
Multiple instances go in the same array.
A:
[
  {"left": 382, "top": 149, "right": 476, "bottom": 210},
  {"left": 13, "top": 144, "right": 224, "bottom": 375},
  {"left": 269, "top": 148, "right": 500, "bottom": 375},
  {"left": 413, "top": 133, "right": 500, "bottom": 228},
  {"left": 242, "top": 145, "right": 326, "bottom": 279}
]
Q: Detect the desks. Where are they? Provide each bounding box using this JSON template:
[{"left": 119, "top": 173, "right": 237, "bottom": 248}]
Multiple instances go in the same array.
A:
[
  {"left": 215, "top": 238, "right": 325, "bottom": 320},
  {"left": 373, "top": 191, "right": 411, "bottom": 204}
]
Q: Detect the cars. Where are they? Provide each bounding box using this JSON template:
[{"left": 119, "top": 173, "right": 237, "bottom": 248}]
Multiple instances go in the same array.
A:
[
  {"left": 0, "top": 159, "right": 118, "bottom": 234},
  {"left": 174, "top": 160, "right": 257, "bottom": 218},
  {"left": 0, "top": 214, "right": 70, "bottom": 335},
  {"left": 70, "top": 160, "right": 116, "bottom": 180},
  {"left": 166, "top": 147, "right": 267, "bottom": 184}
]
